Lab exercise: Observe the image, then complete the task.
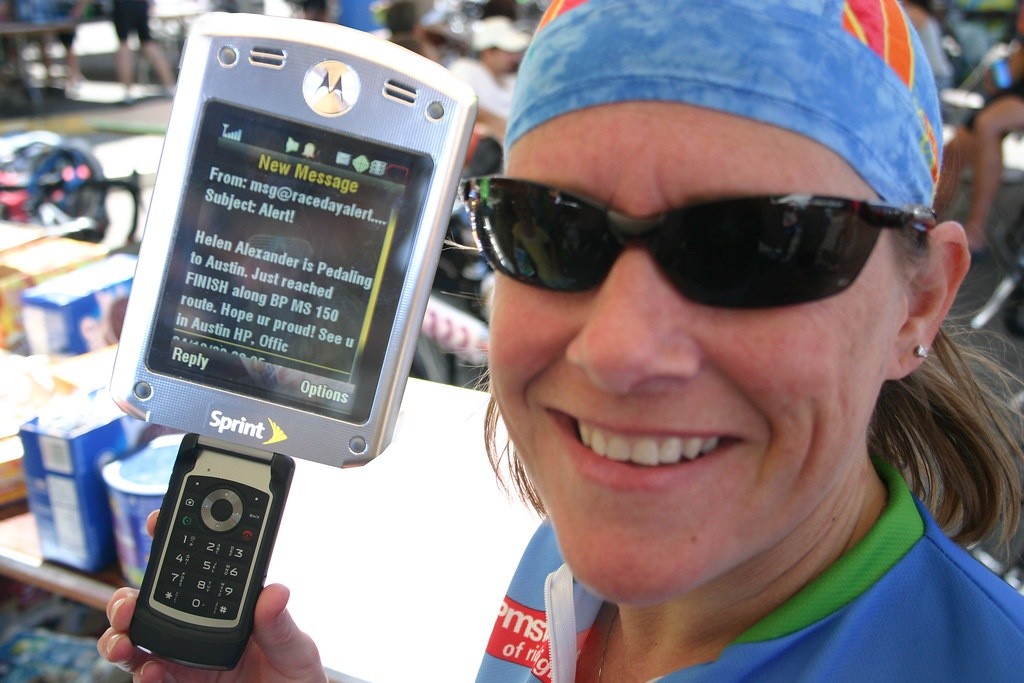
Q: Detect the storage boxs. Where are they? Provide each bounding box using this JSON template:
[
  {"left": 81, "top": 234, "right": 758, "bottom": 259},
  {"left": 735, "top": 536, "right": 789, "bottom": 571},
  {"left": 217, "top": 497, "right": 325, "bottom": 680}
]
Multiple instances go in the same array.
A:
[{"left": 0, "top": 219, "right": 184, "bottom": 681}]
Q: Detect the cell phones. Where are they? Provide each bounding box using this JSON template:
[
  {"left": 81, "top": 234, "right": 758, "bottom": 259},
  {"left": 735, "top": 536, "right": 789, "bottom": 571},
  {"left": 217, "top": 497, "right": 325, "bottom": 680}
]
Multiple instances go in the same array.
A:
[{"left": 107, "top": 13, "right": 478, "bottom": 672}]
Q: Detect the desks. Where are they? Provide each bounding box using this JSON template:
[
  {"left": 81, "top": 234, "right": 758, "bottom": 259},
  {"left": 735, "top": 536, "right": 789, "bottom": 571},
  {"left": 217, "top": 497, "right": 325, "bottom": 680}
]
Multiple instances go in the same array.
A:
[{"left": 0, "top": 375, "right": 552, "bottom": 683}]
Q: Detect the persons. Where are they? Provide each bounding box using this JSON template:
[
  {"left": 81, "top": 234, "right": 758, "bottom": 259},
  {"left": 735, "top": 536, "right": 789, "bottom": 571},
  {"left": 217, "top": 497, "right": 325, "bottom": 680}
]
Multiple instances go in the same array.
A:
[{"left": 0, "top": 0, "right": 1024, "bottom": 683}]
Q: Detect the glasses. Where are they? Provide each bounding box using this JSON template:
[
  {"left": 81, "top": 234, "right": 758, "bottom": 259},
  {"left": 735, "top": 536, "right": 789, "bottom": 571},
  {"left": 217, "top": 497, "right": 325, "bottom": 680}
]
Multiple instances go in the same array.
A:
[{"left": 453, "top": 175, "right": 936, "bottom": 308}]
